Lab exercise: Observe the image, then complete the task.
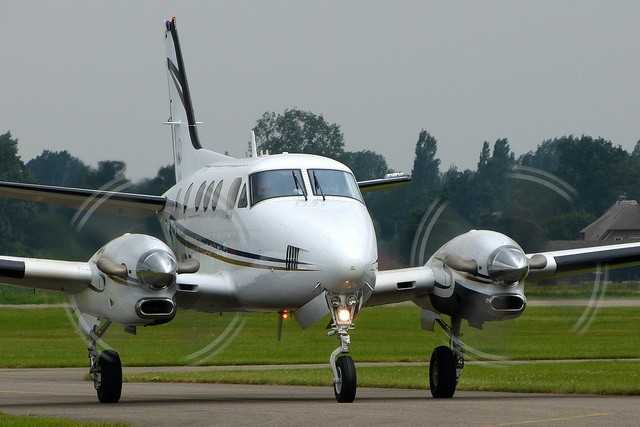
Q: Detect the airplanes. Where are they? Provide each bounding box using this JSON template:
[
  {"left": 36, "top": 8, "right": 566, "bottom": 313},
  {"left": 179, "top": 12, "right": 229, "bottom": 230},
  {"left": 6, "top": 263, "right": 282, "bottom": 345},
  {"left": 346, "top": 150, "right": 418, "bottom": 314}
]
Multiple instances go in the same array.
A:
[{"left": 0, "top": 15, "right": 640, "bottom": 403}]
